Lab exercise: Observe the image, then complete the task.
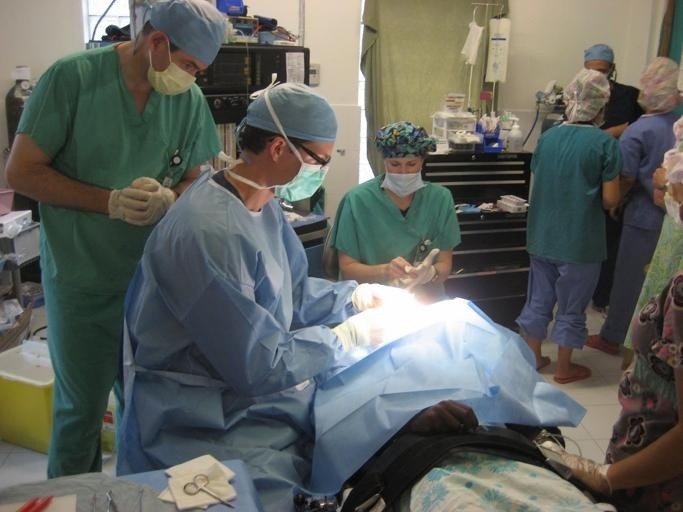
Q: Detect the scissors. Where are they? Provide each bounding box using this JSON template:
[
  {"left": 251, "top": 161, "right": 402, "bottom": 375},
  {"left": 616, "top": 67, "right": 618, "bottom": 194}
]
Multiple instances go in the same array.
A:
[{"left": 184, "top": 474, "right": 236, "bottom": 509}]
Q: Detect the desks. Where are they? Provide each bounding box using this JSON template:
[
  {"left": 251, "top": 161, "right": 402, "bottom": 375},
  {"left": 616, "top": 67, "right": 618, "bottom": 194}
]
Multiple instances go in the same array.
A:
[{"left": 0, "top": 459, "right": 269, "bottom": 512}]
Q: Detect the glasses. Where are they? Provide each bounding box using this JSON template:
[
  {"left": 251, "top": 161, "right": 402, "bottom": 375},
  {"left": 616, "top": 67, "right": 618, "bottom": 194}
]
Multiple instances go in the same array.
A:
[
  {"left": 180, "top": 61, "right": 203, "bottom": 78},
  {"left": 294, "top": 141, "right": 330, "bottom": 169}
]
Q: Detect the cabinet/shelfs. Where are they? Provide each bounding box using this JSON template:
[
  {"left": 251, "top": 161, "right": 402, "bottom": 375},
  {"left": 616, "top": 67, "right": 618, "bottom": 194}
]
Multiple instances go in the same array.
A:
[{"left": 426, "top": 150, "right": 532, "bottom": 332}]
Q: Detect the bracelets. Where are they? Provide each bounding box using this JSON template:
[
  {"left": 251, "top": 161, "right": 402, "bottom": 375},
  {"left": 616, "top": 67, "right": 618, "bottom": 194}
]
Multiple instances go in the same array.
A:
[{"left": 426, "top": 264, "right": 439, "bottom": 288}]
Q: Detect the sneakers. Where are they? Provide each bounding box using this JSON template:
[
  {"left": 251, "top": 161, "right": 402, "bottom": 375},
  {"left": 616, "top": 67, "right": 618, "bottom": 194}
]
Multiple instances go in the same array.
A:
[{"left": 585, "top": 335, "right": 615, "bottom": 355}]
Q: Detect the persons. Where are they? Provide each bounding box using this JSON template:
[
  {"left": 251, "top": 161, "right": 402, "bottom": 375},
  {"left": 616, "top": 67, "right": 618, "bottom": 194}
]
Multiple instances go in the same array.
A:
[
  {"left": 329, "top": 121, "right": 461, "bottom": 303},
  {"left": 5, "top": 0, "right": 227, "bottom": 481},
  {"left": 515, "top": 43, "right": 683, "bottom": 512},
  {"left": 117, "top": 72, "right": 416, "bottom": 512},
  {"left": 375, "top": 399, "right": 601, "bottom": 512}
]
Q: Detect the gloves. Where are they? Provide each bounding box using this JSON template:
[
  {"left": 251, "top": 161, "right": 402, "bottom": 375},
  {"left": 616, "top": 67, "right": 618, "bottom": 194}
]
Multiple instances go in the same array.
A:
[
  {"left": 352, "top": 283, "right": 387, "bottom": 312},
  {"left": 331, "top": 310, "right": 385, "bottom": 351},
  {"left": 107, "top": 177, "right": 175, "bottom": 226},
  {"left": 398, "top": 248, "right": 440, "bottom": 291}
]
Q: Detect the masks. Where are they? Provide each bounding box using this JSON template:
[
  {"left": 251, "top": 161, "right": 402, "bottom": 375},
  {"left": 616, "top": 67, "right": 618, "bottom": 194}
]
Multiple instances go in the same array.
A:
[
  {"left": 273, "top": 150, "right": 329, "bottom": 203},
  {"left": 147, "top": 35, "right": 197, "bottom": 96},
  {"left": 380, "top": 170, "right": 426, "bottom": 198}
]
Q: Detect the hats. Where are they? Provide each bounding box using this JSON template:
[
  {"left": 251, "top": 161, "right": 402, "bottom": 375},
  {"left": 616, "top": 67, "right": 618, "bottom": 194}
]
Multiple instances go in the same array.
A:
[
  {"left": 584, "top": 44, "right": 614, "bottom": 65},
  {"left": 566, "top": 57, "right": 680, "bottom": 123},
  {"left": 374, "top": 121, "right": 436, "bottom": 158},
  {"left": 150, "top": 0, "right": 225, "bottom": 66},
  {"left": 247, "top": 82, "right": 337, "bottom": 142}
]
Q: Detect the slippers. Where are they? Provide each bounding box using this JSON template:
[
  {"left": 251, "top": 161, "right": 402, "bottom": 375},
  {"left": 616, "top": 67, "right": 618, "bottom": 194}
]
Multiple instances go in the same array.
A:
[
  {"left": 554, "top": 365, "right": 591, "bottom": 384},
  {"left": 537, "top": 356, "right": 551, "bottom": 371}
]
